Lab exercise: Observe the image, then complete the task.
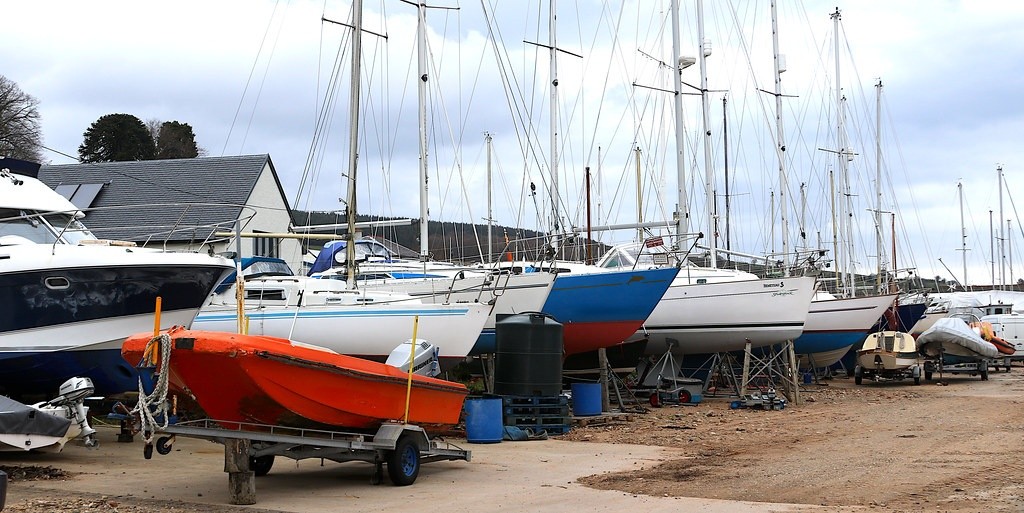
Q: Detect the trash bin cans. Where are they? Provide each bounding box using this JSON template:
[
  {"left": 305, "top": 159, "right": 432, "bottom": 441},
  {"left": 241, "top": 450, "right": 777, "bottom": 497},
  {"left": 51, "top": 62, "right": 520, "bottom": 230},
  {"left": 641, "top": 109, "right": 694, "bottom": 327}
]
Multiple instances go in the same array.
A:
[
  {"left": 571, "top": 381, "right": 602, "bottom": 417},
  {"left": 465, "top": 394, "right": 504, "bottom": 444}
]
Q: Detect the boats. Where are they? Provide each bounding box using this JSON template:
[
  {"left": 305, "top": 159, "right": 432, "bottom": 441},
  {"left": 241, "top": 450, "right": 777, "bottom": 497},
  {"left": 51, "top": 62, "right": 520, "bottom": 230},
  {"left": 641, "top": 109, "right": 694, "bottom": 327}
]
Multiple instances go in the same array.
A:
[
  {"left": 855, "top": 330, "right": 918, "bottom": 370},
  {"left": 969, "top": 321, "right": 1016, "bottom": 357},
  {"left": 103, "top": 295, "right": 471, "bottom": 435},
  {"left": 0, "top": 152, "right": 259, "bottom": 419},
  {"left": 915, "top": 317, "right": 998, "bottom": 365}
]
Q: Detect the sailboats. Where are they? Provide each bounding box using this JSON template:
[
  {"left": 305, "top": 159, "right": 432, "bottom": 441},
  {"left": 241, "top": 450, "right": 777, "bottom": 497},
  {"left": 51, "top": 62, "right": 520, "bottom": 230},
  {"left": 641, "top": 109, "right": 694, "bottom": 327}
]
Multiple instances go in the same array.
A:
[{"left": 184, "top": 0, "right": 1024, "bottom": 385}]
